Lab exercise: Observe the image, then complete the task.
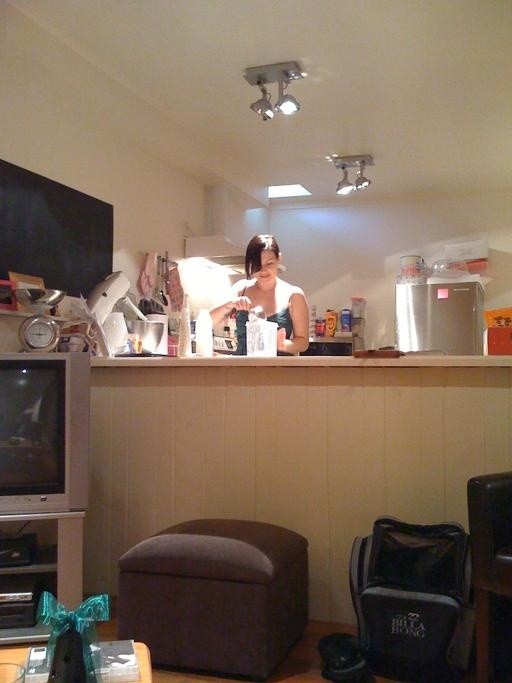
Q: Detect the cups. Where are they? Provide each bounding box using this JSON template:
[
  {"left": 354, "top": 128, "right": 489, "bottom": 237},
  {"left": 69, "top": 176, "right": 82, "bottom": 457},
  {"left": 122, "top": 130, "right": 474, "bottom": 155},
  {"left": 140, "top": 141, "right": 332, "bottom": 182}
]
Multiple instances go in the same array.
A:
[
  {"left": 249, "top": 305, "right": 268, "bottom": 322},
  {"left": 308, "top": 297, "right": 365, "bottom": 336}
]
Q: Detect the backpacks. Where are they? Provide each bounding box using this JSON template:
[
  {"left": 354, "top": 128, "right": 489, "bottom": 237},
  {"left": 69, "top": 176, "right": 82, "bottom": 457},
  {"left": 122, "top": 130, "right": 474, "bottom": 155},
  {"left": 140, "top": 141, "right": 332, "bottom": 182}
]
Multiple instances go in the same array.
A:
[{"left": 345, "top": 512, "right": 471, "bottom": 683}]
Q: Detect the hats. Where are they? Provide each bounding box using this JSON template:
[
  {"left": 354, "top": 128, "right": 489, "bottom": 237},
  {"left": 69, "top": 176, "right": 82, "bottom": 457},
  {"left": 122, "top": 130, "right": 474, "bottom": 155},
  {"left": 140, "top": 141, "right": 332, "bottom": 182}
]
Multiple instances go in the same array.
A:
[{"left": 317, "top": 632, "right": 375, "bottom": 683}]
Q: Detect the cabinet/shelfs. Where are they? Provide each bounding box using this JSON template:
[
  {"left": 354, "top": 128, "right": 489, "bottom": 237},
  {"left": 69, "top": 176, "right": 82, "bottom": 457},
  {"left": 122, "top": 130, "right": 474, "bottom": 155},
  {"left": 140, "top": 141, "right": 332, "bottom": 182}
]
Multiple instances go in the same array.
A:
[{"left": 1, "top": 517, "right": 84, "bottom": 645}]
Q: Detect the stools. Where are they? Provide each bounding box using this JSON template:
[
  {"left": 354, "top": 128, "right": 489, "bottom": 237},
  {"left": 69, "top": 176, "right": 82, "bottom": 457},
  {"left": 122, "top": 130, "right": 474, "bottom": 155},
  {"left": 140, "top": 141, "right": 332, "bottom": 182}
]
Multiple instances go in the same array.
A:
[{"left": 116, "top": 516, "right": 307, "bottom": 679}]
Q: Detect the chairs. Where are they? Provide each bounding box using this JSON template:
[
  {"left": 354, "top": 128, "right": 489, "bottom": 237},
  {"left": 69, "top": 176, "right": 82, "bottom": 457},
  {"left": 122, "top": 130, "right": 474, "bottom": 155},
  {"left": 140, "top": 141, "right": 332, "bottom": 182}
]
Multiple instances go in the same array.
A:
[{"left": 467, "top": 471, "right": 512, "bottom": 683}]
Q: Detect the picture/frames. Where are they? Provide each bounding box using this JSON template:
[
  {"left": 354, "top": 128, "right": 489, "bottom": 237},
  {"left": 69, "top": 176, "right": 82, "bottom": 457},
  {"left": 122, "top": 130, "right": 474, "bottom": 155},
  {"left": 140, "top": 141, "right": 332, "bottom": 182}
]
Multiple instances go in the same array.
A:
[{"left": 0, "top": 279, "right": 17, "bottom": 310}]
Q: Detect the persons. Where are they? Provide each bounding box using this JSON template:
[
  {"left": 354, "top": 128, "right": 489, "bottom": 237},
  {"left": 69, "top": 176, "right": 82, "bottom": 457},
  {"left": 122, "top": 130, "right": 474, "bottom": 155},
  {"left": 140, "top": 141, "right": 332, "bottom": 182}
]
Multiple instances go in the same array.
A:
[{"left": 208, "top": 233, "right": 310, "bottom": 355}]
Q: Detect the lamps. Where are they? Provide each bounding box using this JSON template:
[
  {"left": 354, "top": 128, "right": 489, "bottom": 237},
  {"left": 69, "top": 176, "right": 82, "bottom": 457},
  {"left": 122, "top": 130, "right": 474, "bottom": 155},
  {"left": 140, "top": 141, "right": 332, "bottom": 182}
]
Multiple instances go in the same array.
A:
[
  {"left": 243, "top": 63, "right": 304, "bottom": 120},
  {"left": 332, "top": 155, "right": 375, "bottom": 194}
]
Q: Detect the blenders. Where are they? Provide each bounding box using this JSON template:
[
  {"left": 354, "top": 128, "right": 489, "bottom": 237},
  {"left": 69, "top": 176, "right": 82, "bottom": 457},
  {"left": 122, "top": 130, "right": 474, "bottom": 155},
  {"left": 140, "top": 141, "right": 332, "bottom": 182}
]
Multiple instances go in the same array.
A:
[{"left": 88, "top": 270, "right": 167, "bottom": 356}]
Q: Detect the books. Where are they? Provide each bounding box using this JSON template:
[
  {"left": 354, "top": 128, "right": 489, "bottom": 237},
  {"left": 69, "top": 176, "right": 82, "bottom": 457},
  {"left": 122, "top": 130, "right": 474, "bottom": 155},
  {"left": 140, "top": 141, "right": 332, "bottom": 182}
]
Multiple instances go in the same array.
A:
[{"left": 25, "top": 639, "right": 140, "bottom": 683}]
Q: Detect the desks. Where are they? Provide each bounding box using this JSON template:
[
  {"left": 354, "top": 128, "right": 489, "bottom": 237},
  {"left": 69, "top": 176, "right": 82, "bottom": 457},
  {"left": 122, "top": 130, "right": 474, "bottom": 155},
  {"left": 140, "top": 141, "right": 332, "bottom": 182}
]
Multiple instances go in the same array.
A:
[{"left": 0, "top": 640, "right": 153, "bottom": 683}]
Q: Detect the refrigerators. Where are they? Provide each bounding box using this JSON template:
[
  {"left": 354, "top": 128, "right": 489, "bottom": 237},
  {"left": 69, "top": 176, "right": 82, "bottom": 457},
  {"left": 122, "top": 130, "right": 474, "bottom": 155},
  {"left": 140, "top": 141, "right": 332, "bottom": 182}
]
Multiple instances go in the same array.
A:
[{"left": 393, "top": 279, "right": 486, "bottom": 357}]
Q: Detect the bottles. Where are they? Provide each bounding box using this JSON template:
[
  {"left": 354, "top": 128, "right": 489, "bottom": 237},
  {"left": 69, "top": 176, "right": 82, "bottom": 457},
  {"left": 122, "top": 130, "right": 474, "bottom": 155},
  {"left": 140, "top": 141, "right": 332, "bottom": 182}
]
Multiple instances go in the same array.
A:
[
  {"left": 176, "top": 293, "right": 191, "bottom": 358},
  {"left": 194, "top": 309, "right": 215, "bottom": 355},
  {"left": 234, "top": 307, "right": 249, "bottom": 355}
]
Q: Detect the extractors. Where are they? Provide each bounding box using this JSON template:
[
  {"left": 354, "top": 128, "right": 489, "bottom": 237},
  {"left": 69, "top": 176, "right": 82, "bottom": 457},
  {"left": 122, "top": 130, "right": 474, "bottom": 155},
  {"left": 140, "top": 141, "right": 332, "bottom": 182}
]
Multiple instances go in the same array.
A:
[{"left": 183, "top": 182, "right": 285, "bottom": 276}]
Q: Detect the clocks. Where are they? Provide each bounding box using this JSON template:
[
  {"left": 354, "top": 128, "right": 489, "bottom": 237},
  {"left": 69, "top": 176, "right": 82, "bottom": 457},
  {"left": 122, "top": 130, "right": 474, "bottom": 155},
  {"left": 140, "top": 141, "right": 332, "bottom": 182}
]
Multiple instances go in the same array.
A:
[{"left": 19, "top": 315, "right": 60, "bottom": 352}]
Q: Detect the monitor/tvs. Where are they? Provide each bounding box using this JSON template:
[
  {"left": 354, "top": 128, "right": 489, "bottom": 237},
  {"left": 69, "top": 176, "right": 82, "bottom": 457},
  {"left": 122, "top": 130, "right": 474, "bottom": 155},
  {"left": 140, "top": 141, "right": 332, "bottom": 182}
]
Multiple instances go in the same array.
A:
[{"left": 0, "top": 351, "right": 91, "bottom": 513}]
